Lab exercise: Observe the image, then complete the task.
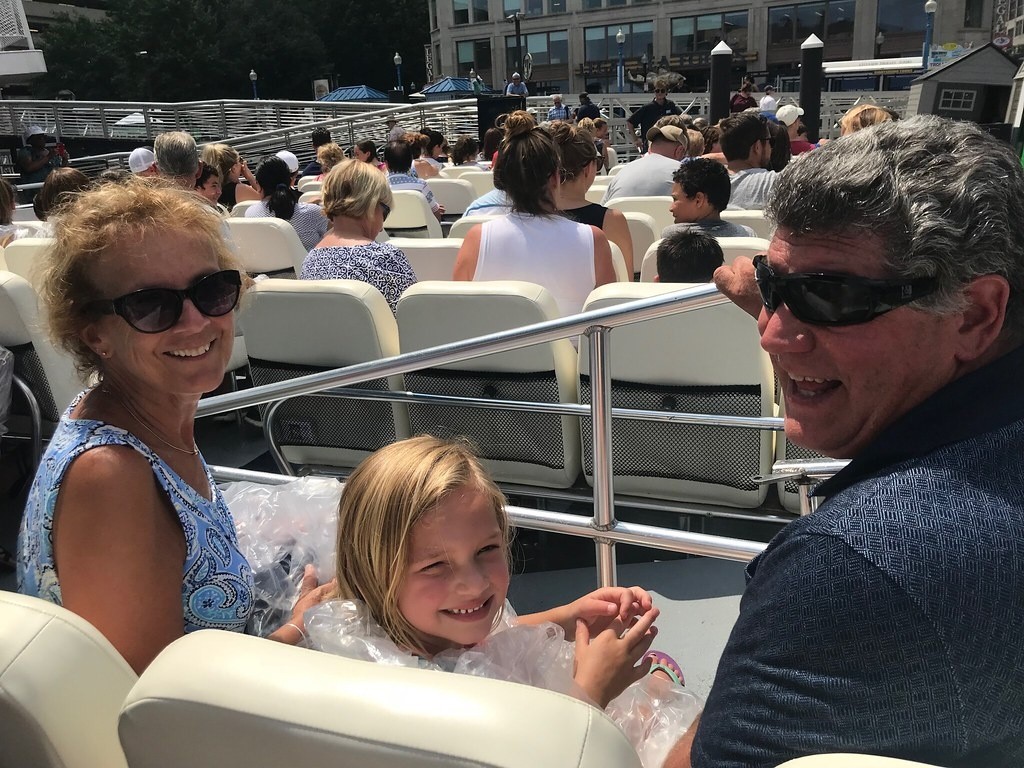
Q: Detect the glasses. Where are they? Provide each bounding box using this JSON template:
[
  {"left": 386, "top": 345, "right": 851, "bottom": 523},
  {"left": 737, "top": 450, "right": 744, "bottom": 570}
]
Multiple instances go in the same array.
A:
[
  {"left": 85, "top": 269, "right": 243, "bottom": 335},
  {"left": 379, "top": 202, "right": 389, "bottom": 220},
  {"left": 584, "top": 156, "right": 605, "bottom": 171},
  {"left": 752, "top": 254, "right": 939, "bottom": 327},
  {"left": 654, "top": 89, "right": 667, "bottom": 94}
]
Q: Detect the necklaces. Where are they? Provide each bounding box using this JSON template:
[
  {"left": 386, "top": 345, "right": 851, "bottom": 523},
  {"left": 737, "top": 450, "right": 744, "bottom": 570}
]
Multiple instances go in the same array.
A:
[{"left": 102, "top": 387, "right": 198, "bottom": 454}]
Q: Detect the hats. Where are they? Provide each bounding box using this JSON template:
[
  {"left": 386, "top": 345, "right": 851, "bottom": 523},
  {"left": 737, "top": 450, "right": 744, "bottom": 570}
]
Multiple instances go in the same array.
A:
[
  {"left": 775, "top": 105, "right": 804, "bottom": 126},
  {"left": 646, "top": 125, "right": 688, "bottom": 150},
  {"left": 764, "top": 85, "right": 777, "bottom": 91},
  {"left": 129, "top": 146, "right": 158, "bottom": 173},
  {"left": 23, "top": 126, "right": 47, "bottom": 142},
  {"left": 275, "top": 150, "right": 300, "bottom": 174}
]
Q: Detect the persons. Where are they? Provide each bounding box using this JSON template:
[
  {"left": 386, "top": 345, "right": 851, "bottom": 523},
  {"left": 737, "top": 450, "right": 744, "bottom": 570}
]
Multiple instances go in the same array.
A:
[
  {"left": 662, "top": 116, "right": 1023, "bottom": 768},
  {"left": 0, "top": 72, "right": 896, "bottom": 316},
  {"left": 17, "top": 184, "right": 336, "bottom": 678},
  {"left": 337, "top": 435, "right": 686, "bottom": 743}
]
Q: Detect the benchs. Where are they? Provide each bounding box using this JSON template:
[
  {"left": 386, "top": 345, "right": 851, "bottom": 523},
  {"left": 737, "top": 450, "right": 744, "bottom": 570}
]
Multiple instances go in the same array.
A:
[{"left": 0, "top": 161, "right": 951, "bottom": 768}]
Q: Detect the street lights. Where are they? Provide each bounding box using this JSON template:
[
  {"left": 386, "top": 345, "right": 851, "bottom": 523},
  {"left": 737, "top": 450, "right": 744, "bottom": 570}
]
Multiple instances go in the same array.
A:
[
  {"left": 873, "top": 32, "right": 885, "bottom": 91},
  {"left": 921, "top": 0, "right": 938, "bottom": 69},
  {"left": 615, "top": 29, "right": 626, "bottom": 118},
  {"left": 249, "top": 69, "right": 258, "bottom": 100},
  {"left": 394, "top": 52, "right": 402, "bottom": 90},
  {"left": 641, "top": 52, "right": 648, "bottom": 91}
]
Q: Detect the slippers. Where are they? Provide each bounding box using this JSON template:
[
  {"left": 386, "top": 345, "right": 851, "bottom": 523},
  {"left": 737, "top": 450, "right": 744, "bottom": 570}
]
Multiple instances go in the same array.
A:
[{"left": 640, "top": 650, "right": 686, "bottom": 691}]
